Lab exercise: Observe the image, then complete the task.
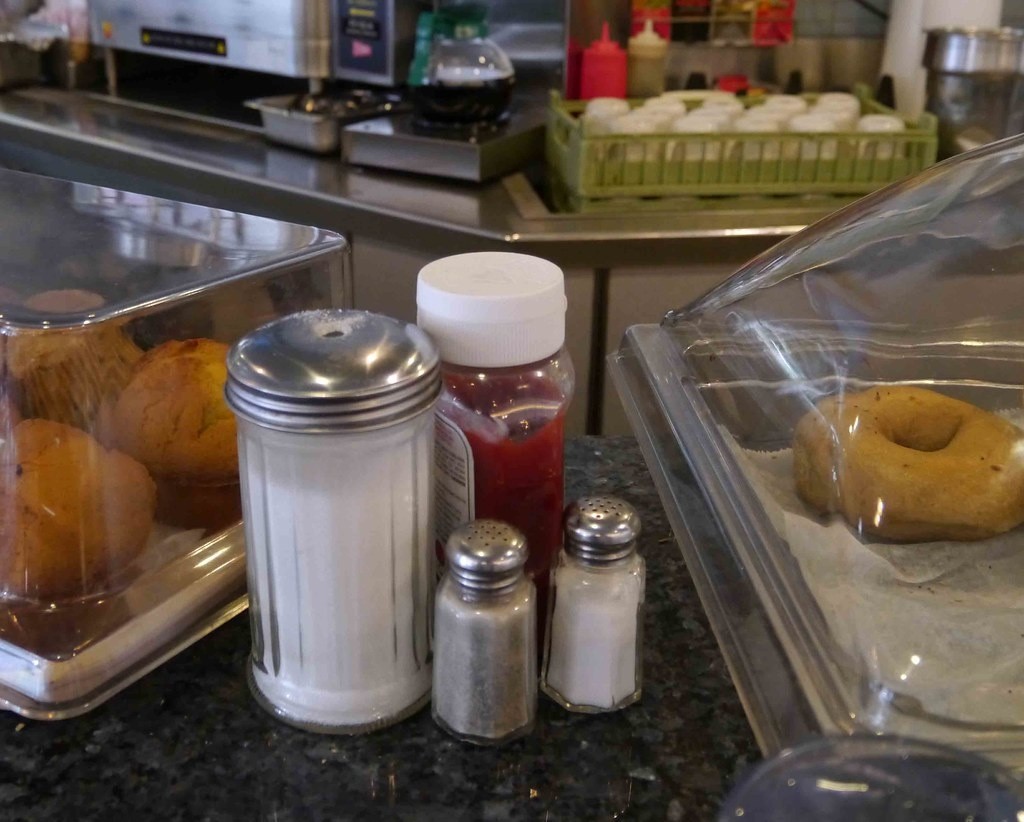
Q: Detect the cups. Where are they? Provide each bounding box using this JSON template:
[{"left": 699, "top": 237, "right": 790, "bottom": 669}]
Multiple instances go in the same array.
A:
[
  {"left": 670, "top": 97, "right": 744, "bottom": 161},
  {"left": 586, "top": 97, "right": 686, "bottom": 162},
  {"left": 857, "top": 115, "right": 907, "bottom": 160},
  {"left": 736, "top": 94, "right": 806, "bottom": 161},
  {"left": 789, "top": 94, "right": 859, "bottom": 162}
]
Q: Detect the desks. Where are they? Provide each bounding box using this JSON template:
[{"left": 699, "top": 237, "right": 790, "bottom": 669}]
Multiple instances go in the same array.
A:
[{"left": 1, "top": 435, "right": 824, "bottom": 821}]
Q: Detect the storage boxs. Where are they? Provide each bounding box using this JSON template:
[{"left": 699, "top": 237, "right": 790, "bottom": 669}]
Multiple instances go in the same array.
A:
[{"left": 546, "top": 82, "right": 937, "bottom": 213}]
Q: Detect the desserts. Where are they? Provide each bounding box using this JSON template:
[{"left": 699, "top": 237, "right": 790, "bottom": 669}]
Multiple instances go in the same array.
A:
[{"left": 0, "top": 290, "right": 241, "bottom": 656}]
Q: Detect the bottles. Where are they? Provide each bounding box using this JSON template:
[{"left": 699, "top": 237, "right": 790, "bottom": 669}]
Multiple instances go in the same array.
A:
[
  {"left": 223, "top": 309, "right": 445, "bottom": 733},
  {"left": 429, "top": 33, "right": 516, "bottom": 127},
  {"left": 626, "top": 20, "right": 670, "bottom": 100},
  {"left": 415, "top": 253, "right": 575, "bottom": 588},
  {"left": 539, "top": 495, "right": 649, "bottom": 715},
  {"left": 580, "top": 20, "right": 627, "bottom": 102},
  {"left": 431, "top": 518, "right": 538, "bottom": 749}
]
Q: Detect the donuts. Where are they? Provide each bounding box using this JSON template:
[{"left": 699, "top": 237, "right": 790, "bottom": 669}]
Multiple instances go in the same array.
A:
[{"left": 791, "top": 386, "right": 1024, "bottom": 543}]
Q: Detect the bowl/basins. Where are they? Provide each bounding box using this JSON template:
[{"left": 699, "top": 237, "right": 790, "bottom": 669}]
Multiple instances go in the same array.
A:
[{"left": 922, "top": 26, "right": 1024, "bottom": 76}]
